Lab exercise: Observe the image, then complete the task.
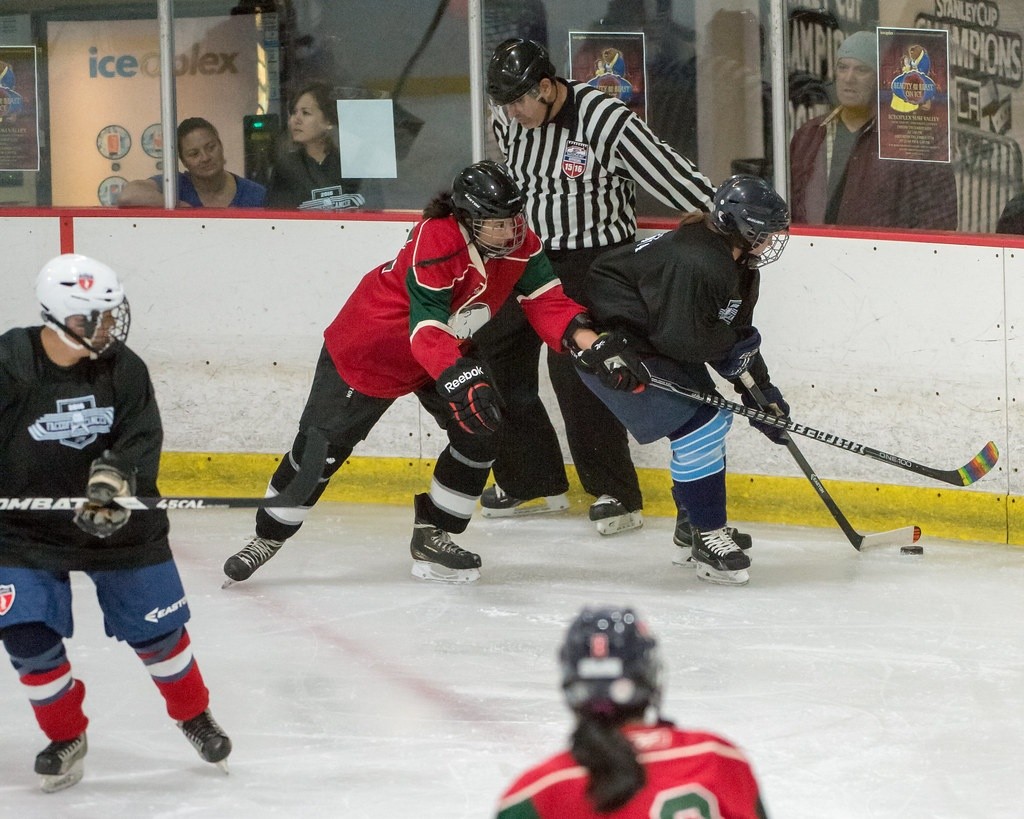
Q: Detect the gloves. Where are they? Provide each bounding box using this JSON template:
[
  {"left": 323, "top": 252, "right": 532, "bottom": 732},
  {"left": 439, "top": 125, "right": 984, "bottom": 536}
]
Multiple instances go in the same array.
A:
[
  {"left": 717, "top": 326, "right": 761, "bottom": 380},
  {"left": 574, "top": 329, "right": 652, "bottom": 393},
  {"left": 741, "top": 383, "right": 791, "bottom": 445},
  {"left": 73, "top": 458, "right": 135, "bottom": 538},
  {"left": 435, "top": 357, "right": 507, "bottom": 435}
]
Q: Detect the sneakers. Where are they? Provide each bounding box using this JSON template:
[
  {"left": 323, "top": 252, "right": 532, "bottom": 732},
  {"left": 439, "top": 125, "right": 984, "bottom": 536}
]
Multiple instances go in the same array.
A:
[
  {"left": 34, "top": 730, "right": 87, "bottom": 793},
  {"left": 671, "top": 486, "right": 752, "bottom": 567},
  {"left": 176, "top": 706, "right": 232, "bottom": 775},
  {"left": 222, "top": 536, "right": 286, "bottom": 589},
  {"left": 480, "top": 483, "right": 570, "bottom": 518},
  {"left": 690, "top": 524, "right": 750, "bottom": 586},
  {"left": 410, "top": 494, "right": 482, "bottom": 584},
  {"left": 589, "top": 494, "right": 643, "bottom": 536}
]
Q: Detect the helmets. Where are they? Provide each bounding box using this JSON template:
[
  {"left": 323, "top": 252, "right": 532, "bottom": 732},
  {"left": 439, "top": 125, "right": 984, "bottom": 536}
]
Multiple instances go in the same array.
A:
[
  {"left": 557, "top": 604, "right": 661, "bottom": 717},
  {"left": 37, "top": 253, "right": 131, "bottom": 361},
  {"left": 712, "top": 174, "right": 791, "bottom": 269},
  {"left": 451, "top": 160, "right": 526, "bottom": 260},
  {"left": 485, "top": 37, "right": 556, "bottom": 105}
]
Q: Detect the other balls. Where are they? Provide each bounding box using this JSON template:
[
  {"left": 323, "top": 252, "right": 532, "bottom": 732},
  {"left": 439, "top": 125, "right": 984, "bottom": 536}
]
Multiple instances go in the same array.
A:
[{"left": 900, "top": 545, "right": 924, "bottom": 556}]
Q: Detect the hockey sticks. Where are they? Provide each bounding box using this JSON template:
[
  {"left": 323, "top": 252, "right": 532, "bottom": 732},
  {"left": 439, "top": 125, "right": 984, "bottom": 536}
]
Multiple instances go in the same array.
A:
[
  {"left": 740, "top": 370, "right": 922, "bottom": 553},
  {"left": 649, "top": 375, "right": 1000, "bottom": 489},
  {"left": 1, "top": 425, "right": 331, "bottom": 513}
]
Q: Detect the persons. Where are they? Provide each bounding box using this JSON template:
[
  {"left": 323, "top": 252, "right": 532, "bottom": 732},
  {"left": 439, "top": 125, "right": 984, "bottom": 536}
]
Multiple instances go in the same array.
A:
[
  {"left": 496, "top": 609, "right": 768, "bottom": 819},
  {"left": 254, "top": 85, "right": 364, "bottom": 209},
  {"left": 116, "top": 118, "right": 267, "bottom": 208},
  {"left": 791, "top": 31, "right": 957, "bottom": 231},
  {"left": 470, "top": 39, "right": 720, "bottom": 535},
  {"left": 634, "top": 7, "right": 834, "bottom": 171},
  {"left": 0, "top": 253, "right": 232, "bottom": 794},
  {"left": 574, "top": 173, "right": 790, "bottom": 586},
  {"left": 219, "top": 162, "right": 651, "bottom": 590}
]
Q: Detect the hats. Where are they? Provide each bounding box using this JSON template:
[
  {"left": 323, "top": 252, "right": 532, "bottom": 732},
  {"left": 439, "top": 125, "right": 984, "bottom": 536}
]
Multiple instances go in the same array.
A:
[{"left": 835, "top": 30, "right": 877, "bottom": 75}]
